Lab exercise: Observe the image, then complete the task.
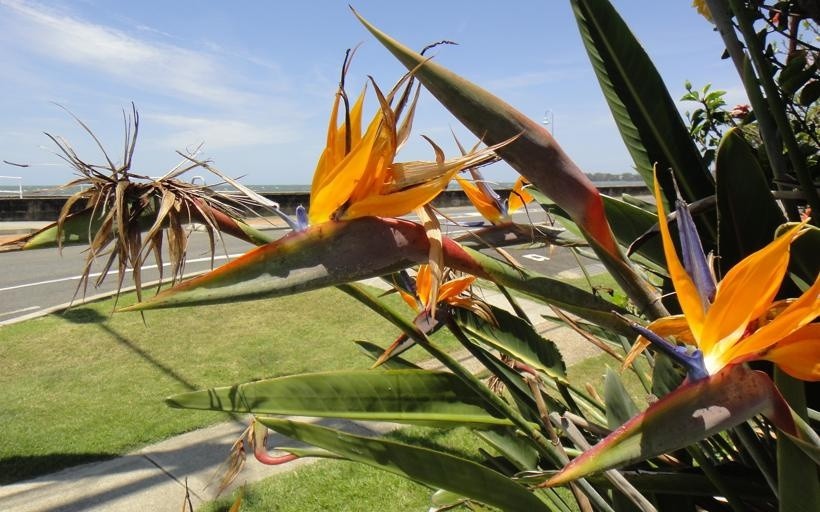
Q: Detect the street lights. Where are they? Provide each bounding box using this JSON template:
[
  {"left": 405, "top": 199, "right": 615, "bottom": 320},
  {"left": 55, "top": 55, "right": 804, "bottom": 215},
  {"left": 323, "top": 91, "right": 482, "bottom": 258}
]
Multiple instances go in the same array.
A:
[{"left": 542, "top": 108, "right": 555, "bottom": 137}]
